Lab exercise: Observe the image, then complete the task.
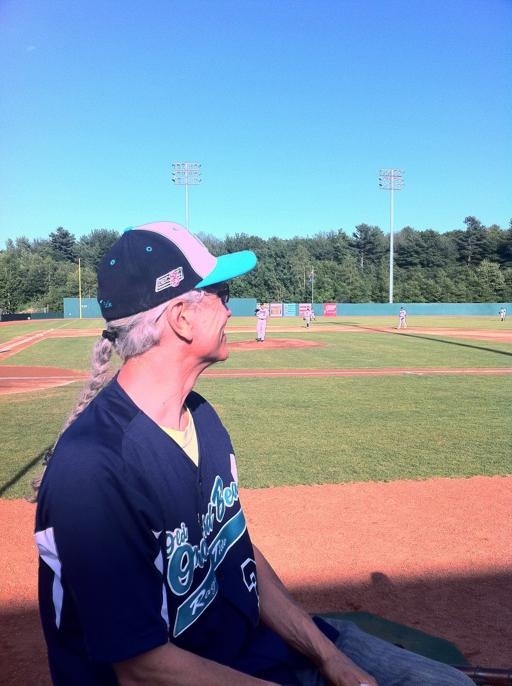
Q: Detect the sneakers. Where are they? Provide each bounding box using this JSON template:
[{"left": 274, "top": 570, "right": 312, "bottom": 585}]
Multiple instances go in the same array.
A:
[{"left": 256, "top": 338, "right": 264, "bottom": 342}]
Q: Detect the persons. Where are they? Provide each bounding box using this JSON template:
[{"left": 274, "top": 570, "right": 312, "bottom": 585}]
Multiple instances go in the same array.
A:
[
  {"left": 311, "top": 312, "right": 315, "bottom": 320},
  {"left": 497, "top": 307, "right": 504, "bottom": 322},
  {"left": 17, "top": 221, "right": 480, "bottom": 686},
  {"left": 502, "top": 308, "right": 506, "bottom": 316},
  {"left": 252, "top": 299, "right": 268, "bottom": 341},
  {"left": 396, "top": 305, "right": 408, "bottom": 329},
  {"left": 301, "top": 307, "right": 311, "bottom": 327}
]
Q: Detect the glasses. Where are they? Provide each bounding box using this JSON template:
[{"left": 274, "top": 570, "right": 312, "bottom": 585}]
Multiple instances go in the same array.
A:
[{"left": 204, "top": 282, "right": 230, "bottom": 304}]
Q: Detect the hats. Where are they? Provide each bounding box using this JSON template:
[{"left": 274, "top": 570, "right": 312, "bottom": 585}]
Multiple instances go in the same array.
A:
[{"left": 97, "top": 220, "right": 259, "bottom": 322}]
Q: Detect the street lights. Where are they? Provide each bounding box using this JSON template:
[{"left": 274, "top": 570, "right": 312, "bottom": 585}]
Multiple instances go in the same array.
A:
[
  {"left": 377, "top": 167, "right": 404, "bottom": 304},
  {"left": 172, "top": 161, "right": 204, "bottom": 236}
]
